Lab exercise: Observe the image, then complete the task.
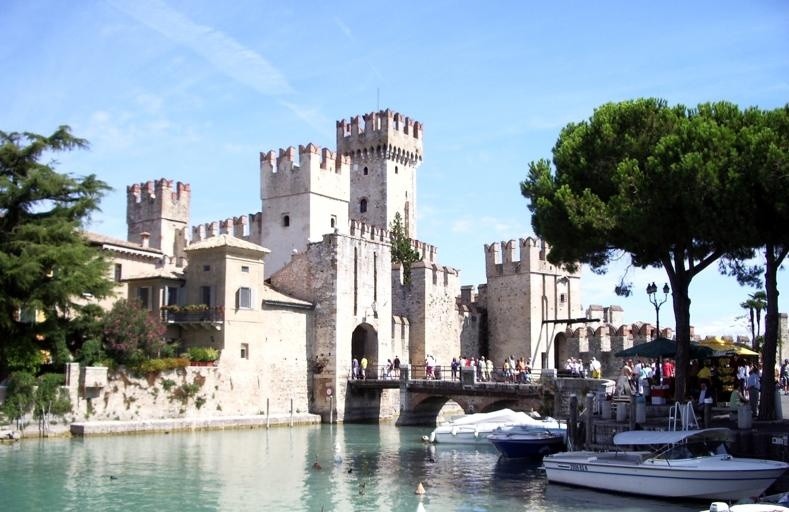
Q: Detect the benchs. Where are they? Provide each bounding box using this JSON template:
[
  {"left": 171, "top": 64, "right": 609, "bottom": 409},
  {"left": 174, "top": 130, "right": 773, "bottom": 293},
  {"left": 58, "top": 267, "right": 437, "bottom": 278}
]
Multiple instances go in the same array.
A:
[{"left": 557, "top": 369, "right": 587, "bottom": 378}]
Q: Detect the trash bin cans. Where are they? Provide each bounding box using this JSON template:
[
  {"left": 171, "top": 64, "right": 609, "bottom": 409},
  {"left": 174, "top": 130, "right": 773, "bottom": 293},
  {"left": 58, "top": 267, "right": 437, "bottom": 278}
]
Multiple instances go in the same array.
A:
[
  {"left": 737, "top": 405, "right": 752, "bottom": 429},
  {"left": 635, "top": 397, "right": 646, "bottom": 423},
  {"left": 616, "top": 403, "right": 626, "bottom": 422},
  {"left": 602, "top": 401, "right": 611, "bottom": 418}
]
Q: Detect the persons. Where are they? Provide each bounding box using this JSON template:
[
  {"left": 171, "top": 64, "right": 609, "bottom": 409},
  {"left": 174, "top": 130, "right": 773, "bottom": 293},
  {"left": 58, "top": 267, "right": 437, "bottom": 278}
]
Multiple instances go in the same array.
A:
[
  {"left": 618, "top": 356, "right": 789, "bottom": 419},
  {"left": 352, "top": 353, "right": 604, "bottom": 386}
]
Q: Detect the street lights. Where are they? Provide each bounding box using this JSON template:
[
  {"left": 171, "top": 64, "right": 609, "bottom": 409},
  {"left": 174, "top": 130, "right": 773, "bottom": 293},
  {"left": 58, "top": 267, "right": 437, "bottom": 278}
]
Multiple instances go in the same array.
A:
[{"left": 645, "top": 281, "right": 669, "bottom": 385}]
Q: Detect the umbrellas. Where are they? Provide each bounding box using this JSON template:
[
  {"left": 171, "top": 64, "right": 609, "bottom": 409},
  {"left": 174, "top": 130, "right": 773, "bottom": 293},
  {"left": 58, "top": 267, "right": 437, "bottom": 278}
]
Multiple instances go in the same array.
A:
[
  {"left": 614, "top": 334, "right": 712, "bottom": 387},
  {"left": 693, "top": 335, "right": 761, "bottom": 359}
]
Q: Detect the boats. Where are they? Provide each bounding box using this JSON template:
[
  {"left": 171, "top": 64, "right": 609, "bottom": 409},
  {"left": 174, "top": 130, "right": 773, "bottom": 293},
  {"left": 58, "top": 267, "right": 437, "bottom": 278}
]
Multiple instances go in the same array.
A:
[
  {"left": 540, "top": 400, "right": 787, "bottom": 501},
  {"left": 431, "top": 402, "right": 565, "bottom": 451}
]
